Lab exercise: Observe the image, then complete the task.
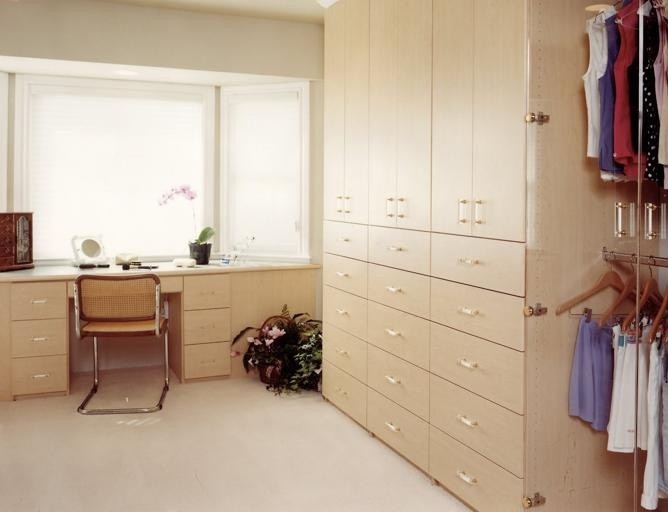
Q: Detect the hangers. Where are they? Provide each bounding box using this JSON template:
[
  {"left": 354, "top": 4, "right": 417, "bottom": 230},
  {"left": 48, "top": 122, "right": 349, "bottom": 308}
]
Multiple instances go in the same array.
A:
[
  {"left": 551, "top": 242, "right": 668, "bottom": 353},
  {"left": 578, "top": 3, "right": 666, "bottom": 37}
]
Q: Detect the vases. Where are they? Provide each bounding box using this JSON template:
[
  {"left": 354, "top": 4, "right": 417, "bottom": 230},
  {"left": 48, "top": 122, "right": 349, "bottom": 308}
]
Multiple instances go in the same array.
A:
[
  {"left": 189, "top": 240, "right": 211, "bottom": 265},
  {"left": 256, "top": 359, "right": 281, "bottom": 385}
]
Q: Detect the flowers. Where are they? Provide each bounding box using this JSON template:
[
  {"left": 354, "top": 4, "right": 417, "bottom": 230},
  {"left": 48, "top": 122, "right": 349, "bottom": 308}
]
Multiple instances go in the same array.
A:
[
  {"left": 156, "top": 182, "right": 215, "bottom": 244},
  {"left": 229, "top": 305, "right": 323, "bottom": 397}
]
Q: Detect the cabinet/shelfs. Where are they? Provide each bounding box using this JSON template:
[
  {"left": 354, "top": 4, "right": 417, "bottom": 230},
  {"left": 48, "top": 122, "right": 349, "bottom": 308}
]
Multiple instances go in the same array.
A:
[
  {"left": 318, "top": 0, "right": 637, "bottom": 511},
  {"left": 0, "top": 254, "right": 322, "bottom": 401}
]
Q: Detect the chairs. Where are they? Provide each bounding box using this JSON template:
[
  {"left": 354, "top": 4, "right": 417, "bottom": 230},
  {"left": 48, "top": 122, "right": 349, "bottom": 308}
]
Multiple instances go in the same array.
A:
[{"left": 70, "top": 273, "right": 173, "bottom": 415}]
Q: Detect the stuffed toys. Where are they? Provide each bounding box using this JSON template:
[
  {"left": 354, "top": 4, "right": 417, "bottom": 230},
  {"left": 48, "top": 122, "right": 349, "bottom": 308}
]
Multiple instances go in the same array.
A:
[{"left": 257, "top": 315, "right": 294, "bottom": 359}]
